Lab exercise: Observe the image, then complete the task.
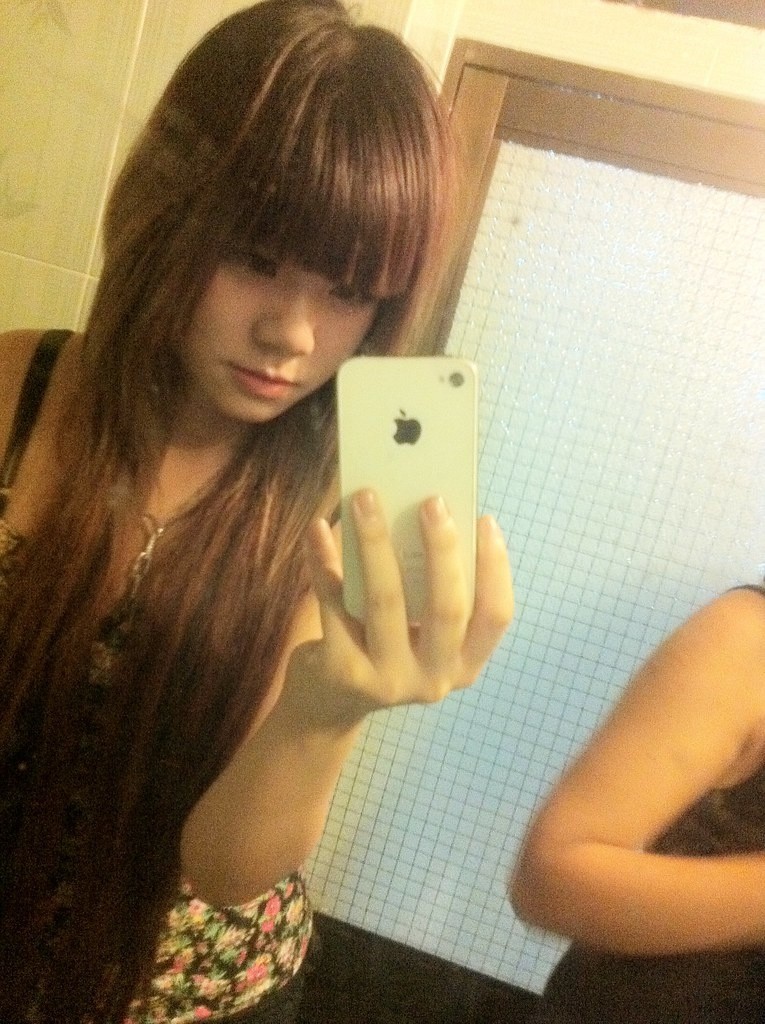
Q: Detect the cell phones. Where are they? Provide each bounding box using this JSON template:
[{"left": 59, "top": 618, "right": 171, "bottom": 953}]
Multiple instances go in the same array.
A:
[{"left": 336, "top": 356, "right": 478, "bottom": 624}]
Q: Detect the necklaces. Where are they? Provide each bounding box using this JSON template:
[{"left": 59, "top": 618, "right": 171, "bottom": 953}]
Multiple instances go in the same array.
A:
[{"left": 111, "top": 456, "right": 228, "bottom": 610}]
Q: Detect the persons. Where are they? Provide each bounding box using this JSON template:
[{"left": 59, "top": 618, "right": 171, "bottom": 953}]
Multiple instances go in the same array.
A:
[
  {"left": 0, "top": 1, "right": 514, "bottom": 1024},
  {"left": 509, "top": 575, "right": 764, "bottom": 1023}
]
